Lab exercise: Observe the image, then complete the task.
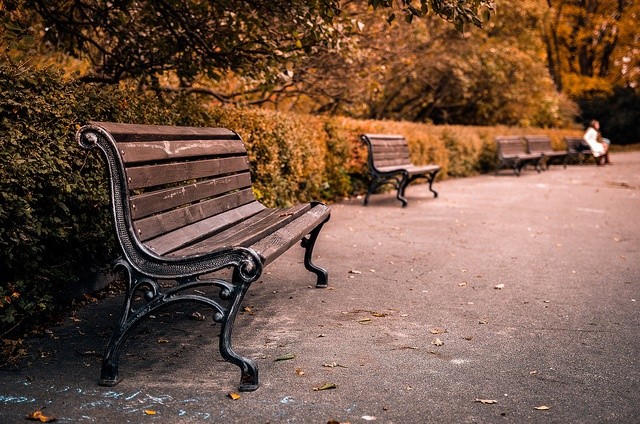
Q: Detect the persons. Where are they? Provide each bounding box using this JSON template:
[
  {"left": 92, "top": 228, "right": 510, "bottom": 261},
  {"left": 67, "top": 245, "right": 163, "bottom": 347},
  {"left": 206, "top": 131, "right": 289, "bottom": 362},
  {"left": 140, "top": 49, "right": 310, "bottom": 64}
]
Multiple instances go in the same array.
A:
[{"left": 583, "top": 119, "right": 612, "bottom": 165}]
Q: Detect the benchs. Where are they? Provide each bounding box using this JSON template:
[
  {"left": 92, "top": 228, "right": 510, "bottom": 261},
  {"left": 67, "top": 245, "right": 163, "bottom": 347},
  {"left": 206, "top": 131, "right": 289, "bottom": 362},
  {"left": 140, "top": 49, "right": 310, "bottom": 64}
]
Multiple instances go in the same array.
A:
[
  {"left": 523, "top": 135, "right": 570, "bottom": 171},
  {"left": 75, "top": 120, "right": 331, "bottom": 393},
  {"left": 563, "top": 136, "right": 593, "bottom": 165},
  {"left": 495, "top": 135, "right": 543, "bottom": 176},
  {"left": 360, "top": 134, "right": 442, "bottom": 208}
]
